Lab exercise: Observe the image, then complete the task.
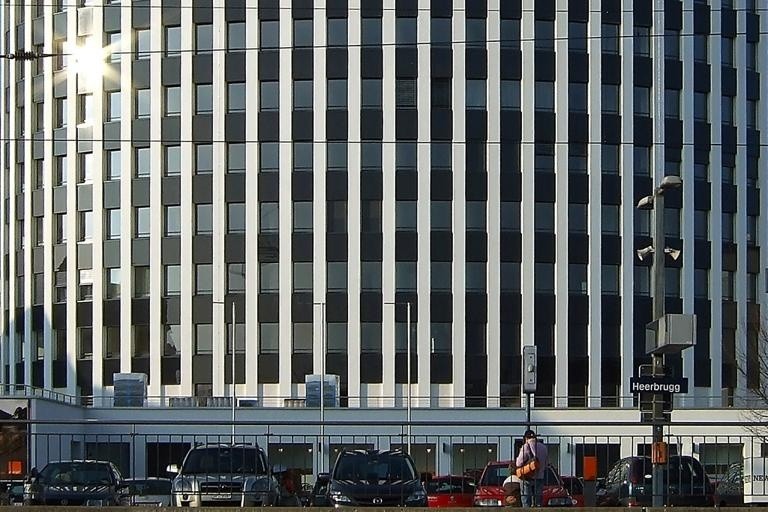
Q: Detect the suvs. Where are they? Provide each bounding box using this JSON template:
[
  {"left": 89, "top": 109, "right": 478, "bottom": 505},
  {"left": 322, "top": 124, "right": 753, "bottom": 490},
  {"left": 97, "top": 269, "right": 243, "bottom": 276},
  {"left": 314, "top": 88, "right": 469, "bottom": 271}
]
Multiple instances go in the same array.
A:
[{"left": 167, "top": 442, "right": 286, "bottom": 507}]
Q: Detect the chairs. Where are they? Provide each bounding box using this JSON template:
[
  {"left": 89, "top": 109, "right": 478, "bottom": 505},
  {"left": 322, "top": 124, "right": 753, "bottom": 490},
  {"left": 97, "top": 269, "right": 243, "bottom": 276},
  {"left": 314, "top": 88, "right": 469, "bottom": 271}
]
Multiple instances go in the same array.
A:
[
  {"left": 340, "top": 463, "right": 365, "bottom": 481},
  {"left": 54, "top": 473, "right": 72, "bottom": 482},
  {"left": 90, "top": 472, "right": 109, "bottom": 483},
  {"left": 386, "top": 462, "right": 408, "bottom": 481}
]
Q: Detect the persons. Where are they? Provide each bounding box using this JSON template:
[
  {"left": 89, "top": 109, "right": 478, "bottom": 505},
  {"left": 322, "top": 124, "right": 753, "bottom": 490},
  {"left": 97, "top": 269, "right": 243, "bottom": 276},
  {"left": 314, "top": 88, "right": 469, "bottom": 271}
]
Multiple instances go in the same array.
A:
[{"left": 515, "top": 429, "right": 548, "bottom": 507}]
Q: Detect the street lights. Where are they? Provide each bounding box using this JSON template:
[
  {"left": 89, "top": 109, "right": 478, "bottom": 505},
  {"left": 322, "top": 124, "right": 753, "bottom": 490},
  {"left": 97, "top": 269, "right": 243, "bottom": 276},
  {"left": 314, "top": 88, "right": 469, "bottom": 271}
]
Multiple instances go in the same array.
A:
[{"left": 636, "top": 176, "right": 683, "bottom": 506}]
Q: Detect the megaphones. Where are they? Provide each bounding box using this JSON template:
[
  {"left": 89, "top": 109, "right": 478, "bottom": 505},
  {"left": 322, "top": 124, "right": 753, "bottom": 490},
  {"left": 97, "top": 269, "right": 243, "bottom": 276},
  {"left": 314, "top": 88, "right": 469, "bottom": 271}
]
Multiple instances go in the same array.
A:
[
  {"left": 667, "top": 246, "right": 682, "bottom": 261},
  {"left": 637, "top": 245, "right": 652, "bottom": 261}
]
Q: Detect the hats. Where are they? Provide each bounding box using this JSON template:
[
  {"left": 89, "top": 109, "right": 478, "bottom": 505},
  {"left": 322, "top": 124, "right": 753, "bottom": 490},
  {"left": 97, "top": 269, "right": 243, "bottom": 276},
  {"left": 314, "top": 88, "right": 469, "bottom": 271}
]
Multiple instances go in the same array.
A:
[{"left": 525, "top": 430, "right": 535, "bottom": 436}]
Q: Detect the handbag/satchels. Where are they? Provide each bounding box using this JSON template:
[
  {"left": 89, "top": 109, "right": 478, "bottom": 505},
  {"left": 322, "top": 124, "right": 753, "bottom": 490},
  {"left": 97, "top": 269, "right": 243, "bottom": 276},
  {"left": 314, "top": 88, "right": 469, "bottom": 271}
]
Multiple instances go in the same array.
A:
[{"left": 515, "top": 456, "right": 539, "bottom": 479}]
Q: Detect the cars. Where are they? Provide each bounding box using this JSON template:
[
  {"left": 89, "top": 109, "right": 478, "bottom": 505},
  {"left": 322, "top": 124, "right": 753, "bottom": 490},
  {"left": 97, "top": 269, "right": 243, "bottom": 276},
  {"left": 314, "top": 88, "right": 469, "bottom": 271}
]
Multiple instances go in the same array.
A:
[
  {"left": 595, "top": 454, "right": 715, "bottom": 508},
  {"left": 473, "top": 459, "right": 572, "bottom": 507},
  {"left": 318, "top": 449, "right": 429, "bottom": 507},
  {"left": 22, "top": 460, "right": 131, "bottom": 507},
  {"left": 715, "top": 462, "right": 745, "bottom": 508},
  {"left": 10, "top": 474, "right": 588, "bottom": 510}
]
